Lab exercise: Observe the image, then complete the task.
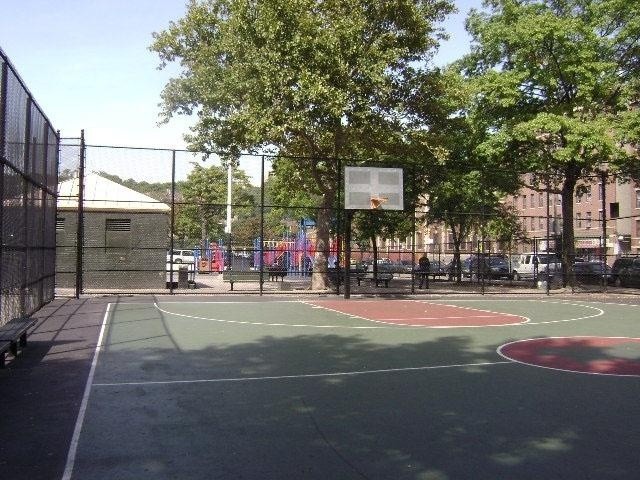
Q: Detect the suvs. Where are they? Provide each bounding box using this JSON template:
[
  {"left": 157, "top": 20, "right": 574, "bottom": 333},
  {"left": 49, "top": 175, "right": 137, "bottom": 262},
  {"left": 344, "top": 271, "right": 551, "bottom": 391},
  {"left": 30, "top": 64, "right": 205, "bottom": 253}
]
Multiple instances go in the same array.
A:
[{"left": 609, "top": 256, "right": 640, "bottom": 288}]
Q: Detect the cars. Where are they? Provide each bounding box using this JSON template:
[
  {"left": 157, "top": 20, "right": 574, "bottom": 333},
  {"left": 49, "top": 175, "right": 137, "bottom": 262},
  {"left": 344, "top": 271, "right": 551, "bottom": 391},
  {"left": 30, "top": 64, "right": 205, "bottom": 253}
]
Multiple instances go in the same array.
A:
[
  {"left": 166, "top": 250, "right": 256, "bottom": 264},
  {"left": 350, "top": 252, "right": 612, "bottom": 287}
]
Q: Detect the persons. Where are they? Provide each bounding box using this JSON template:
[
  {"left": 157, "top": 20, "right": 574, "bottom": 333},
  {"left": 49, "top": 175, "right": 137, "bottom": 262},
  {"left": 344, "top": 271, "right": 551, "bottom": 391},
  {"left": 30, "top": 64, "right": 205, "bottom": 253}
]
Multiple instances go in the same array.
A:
[{"left": 418, "top": 253, "right": 430, "bottom": 289}]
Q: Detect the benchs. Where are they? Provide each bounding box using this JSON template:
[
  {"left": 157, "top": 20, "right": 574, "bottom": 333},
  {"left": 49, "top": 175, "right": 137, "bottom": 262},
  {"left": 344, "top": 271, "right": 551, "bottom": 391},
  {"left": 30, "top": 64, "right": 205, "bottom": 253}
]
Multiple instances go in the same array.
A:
[
  {"left": 357, "top": 273, "right": 392, "bottom": 288},
  {"left": 0, "top": 317, "right": 39, "bottom": 368},
  {"left": 222, "top": 270, "right": 269, "bottom": 290},
  {"left": 266, "top": 267, "right": 287, "bottom": 281}
]
